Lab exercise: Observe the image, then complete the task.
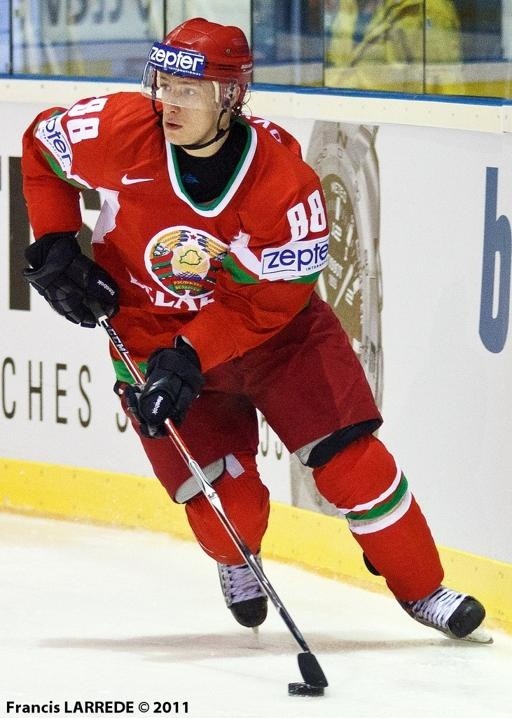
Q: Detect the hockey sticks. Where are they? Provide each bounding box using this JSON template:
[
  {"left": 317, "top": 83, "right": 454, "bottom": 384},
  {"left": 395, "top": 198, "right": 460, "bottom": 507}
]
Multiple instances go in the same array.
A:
[{"left": 86, "top": 303, "right": 331, "bottom": 686}]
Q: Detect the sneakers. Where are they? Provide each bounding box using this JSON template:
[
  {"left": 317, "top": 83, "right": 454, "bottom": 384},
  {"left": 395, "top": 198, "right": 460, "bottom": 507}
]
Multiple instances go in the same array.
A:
[
  {"left": 395, "top": 585, "right": 487, "bottom": 638},
  {"left": 216, "top": 556, "right": 267, "bottom": 627}
]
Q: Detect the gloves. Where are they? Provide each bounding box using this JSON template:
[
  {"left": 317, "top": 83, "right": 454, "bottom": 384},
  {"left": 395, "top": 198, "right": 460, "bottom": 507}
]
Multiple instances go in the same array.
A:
[
  {"left": 21, "top": 231, "right": 121, "bottom": 327},
  {"left": 115, "top": 334, "right": 203, "bottom": 442}
]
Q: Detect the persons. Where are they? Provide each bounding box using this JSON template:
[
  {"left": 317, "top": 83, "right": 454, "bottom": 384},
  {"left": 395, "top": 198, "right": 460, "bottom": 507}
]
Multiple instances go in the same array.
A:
[
  {"left": 323, "top": 0, "right": 472, "bottom": 98},
  {"left": 19, "top": 19, "right": 487, "bottom": 639}
]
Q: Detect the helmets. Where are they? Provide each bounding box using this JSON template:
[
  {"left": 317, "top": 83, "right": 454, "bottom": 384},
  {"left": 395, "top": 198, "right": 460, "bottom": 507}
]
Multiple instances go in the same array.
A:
[{"left": 139, "top": 18, "right": 252, "bottom": 111}]
